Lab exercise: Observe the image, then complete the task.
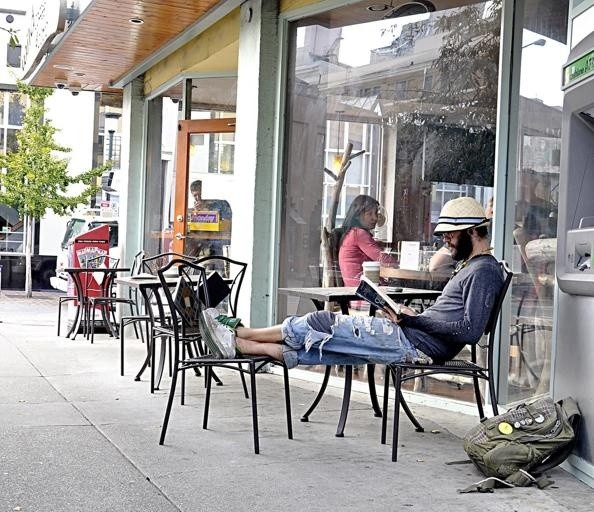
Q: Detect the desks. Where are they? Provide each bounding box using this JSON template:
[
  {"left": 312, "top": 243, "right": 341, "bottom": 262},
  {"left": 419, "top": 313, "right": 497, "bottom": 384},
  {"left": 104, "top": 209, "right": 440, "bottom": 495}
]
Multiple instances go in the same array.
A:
[
  {"left": 115, "top": 272, "right": 235, "bottom": 389},
  {"left": 272, "top": 284, "right": 444, "bottom": 438}
]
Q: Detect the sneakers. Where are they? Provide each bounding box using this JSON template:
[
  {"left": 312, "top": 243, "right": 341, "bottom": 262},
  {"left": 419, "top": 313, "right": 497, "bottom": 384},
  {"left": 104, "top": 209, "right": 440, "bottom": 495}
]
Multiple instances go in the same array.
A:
[{"left": 199, "top": 307, "right": 245, "bottom": 359}]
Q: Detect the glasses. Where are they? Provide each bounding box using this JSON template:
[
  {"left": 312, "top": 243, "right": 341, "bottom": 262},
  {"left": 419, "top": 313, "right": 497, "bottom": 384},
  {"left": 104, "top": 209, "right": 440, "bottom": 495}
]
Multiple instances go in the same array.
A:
[{"left": 441, "top": 231, "right": 455, "bottom": 238}]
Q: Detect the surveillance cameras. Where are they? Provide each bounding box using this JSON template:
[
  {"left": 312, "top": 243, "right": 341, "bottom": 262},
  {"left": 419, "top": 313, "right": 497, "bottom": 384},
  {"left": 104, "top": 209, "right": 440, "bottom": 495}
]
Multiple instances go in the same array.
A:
[
  {"left": 69, "top": 83, "right": 81, "bottom": 96},
  {"left": 54, "top": 79, "right": 67, "bottom": 90}
]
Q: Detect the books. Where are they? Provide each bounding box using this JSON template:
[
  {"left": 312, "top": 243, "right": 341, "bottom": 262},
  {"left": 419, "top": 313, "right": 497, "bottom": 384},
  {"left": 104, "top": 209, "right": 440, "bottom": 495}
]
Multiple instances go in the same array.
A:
[{"left": 355, "top": 275, "right": 401, "bottom": 315}]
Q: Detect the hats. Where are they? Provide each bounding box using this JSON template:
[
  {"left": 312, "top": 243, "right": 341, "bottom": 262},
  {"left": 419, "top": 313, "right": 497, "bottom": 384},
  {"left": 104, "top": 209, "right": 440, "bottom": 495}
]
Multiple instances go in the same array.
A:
[{"left": 434, "top": 196, "right": 491, "bottom": 236}]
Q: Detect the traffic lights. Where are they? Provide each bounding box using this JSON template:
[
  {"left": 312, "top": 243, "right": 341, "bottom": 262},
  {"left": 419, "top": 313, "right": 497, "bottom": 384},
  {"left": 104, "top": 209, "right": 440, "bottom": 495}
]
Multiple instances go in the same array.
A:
[{"left": 101, "top": 172, "right": 117, "bottom": 192}]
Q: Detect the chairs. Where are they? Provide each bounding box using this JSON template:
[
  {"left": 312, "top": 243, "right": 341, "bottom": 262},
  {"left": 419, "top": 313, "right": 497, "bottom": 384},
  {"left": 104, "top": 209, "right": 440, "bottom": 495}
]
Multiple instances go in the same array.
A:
[
  {"left": 59, "top": 251, "right": 146, "bottom": 341},
  {"left": 116, "top": 251, "right": 199, "bottom": 377},
  {"left": 379, "top": 260, "right": 515, "bottom": 462},
  {"left": 513, "top": 226, "right": 554, "bottom": 398},
  {"left": 151, "top": 255, "right": 250, "bottom": 395},
  {"left": 155, "top": 259, "right": 292, "bottom": 454}
]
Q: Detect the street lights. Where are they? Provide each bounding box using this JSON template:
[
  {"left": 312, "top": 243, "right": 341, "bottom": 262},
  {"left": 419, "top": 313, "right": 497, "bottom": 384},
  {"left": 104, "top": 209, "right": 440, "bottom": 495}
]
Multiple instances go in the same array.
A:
[
  {"left": 103, "top": 111, "right": 122, "bottom": 202},
  {"left": 516, "top": 38, "right": 546, "bottom": 202}
]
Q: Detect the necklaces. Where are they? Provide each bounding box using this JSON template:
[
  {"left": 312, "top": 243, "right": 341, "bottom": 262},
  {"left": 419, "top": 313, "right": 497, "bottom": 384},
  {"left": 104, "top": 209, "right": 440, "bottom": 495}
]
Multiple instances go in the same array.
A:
[{"left": 450, "top": 247, "right": 493, "bottom": 279}]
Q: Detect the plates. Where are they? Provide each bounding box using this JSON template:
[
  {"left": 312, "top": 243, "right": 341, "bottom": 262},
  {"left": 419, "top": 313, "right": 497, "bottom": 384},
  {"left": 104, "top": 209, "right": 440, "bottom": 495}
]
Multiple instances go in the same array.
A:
[{"left": 162, "top": 273, "right": 179, "bottom": 279}]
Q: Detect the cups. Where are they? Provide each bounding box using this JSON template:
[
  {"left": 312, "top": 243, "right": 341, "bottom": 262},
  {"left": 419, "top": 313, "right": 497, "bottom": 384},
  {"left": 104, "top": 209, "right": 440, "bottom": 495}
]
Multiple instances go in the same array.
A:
[
  {"left": 423, "top": 246, "right": 437, "bottom": 273},
  {"left": 362, "top": 260, "right": 380, "bottom": 288}
]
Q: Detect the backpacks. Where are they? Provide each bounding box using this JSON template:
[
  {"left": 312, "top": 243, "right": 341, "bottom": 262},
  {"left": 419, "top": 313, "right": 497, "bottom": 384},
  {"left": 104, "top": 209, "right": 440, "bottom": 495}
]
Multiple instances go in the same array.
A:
[{"left": 445, "top": 396, "right": 582, "bottom": 494}]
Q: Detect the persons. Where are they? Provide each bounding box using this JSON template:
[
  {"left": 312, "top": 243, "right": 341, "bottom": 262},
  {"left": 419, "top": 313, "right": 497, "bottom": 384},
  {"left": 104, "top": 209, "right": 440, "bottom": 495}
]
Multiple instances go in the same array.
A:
[
  {"left": 338, "top": 195, "right": 397, "bottom": 287},
  {"left": 199, "top": 197, "right": 504, "bottom": 369},
  {"left": 190, "top": 180, "right": 232, "bottom": 277}
]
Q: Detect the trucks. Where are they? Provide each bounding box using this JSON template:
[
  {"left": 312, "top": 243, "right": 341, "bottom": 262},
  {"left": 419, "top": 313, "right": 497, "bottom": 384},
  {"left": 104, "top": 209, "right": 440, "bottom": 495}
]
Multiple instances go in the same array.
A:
[{"left": 48, "top": 216, "right": 119, "bottom": 295}]
